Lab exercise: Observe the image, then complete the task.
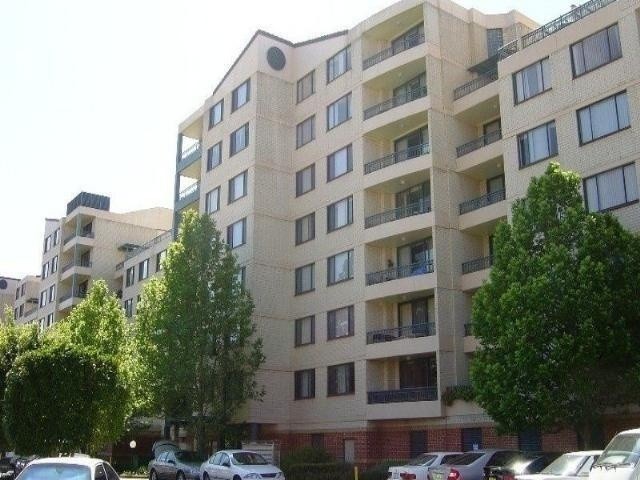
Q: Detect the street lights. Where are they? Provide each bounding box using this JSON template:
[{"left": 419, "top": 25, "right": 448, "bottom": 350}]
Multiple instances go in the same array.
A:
[{"left": 130, "top": 440, "right": 137, "bottom": 475}]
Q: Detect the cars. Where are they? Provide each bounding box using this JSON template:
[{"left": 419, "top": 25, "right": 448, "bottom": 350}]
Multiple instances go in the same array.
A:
[
  {"left": 0, "top": 450, "right": 90, "bottom": 480},
  {"left": 199, "top": 449, "right": 286, "bottom": 480},
  {"left": 14, "top": 457, "right": 120, "bottom": 480},
  {"left": 147, "top": 439, "right": 203, "bottom": 479},
  {"left": 483, "top": 452, "right": 571, "bottom": 480},
  {"left": 388, "top": 451, "right": 464, "bottom": 480},
  {"left": 427, "top": 448, "right": 524, "bottom": 480},
  {"left": 589, "top": 428, "right": 640, "bottom": 480},
  {"left": 514, "top": 450, "right": 640, "bottom": 480}
]
